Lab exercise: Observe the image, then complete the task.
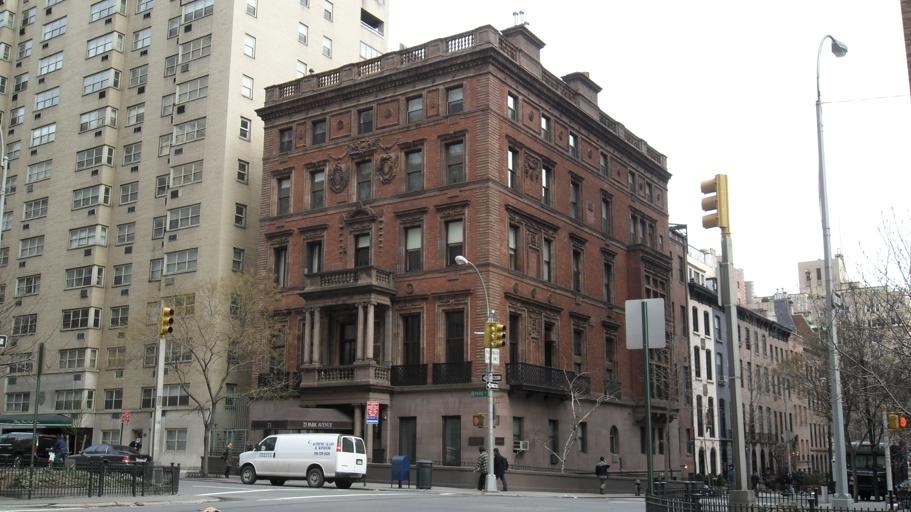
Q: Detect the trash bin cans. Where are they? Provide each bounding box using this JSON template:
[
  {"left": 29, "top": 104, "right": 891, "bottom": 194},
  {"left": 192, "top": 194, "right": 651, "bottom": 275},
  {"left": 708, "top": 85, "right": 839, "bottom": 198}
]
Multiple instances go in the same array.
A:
[
  {"left": 164, "top": 467, "right": 179, "bottom": 494},
  {"left": 415, "top": 461, "right": 433, "bottom": 489},
  {"left": 391, "top": 456, "right": 410, "bottom": 489}
]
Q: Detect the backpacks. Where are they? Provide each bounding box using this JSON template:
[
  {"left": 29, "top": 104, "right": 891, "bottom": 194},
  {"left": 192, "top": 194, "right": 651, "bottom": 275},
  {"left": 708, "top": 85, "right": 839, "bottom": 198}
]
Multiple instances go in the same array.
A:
[{"left": 500, "top": 455, "right": 509, "bottom": 471}]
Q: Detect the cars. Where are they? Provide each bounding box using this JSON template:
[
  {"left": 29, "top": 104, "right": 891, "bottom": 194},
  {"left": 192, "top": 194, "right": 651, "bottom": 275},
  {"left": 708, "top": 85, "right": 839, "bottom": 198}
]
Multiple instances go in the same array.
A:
[{"left": 68, "top": 444, "right": 152, "bottom": 476}]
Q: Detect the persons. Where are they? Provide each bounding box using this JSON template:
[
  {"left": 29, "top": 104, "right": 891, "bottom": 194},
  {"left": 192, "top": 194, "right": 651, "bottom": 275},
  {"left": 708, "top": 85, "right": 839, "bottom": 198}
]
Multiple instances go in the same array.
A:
[
  {"left": 594, "top": 456, "right": 611, "bottom": 495},
  {"left": 224, "top": 442, "right": 233, "bottom": 478},
  {"left": 54, "top": 437, "right": 68, "bottom": 467},
  {"left": 476, "top": 446, "right": 489, "bottom": 492},
  {"left": 493, "top": 447, "right": 508, "bottom": 492},
  {"left": 129, "top": 435, "right": 143, "bottom": 458}
]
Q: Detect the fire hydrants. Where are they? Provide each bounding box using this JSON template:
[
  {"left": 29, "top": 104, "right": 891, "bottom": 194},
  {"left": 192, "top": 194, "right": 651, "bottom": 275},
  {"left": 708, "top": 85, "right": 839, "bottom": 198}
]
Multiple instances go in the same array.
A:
[{"left": 634, "top": 480, "right": 641, "bottom": 496}]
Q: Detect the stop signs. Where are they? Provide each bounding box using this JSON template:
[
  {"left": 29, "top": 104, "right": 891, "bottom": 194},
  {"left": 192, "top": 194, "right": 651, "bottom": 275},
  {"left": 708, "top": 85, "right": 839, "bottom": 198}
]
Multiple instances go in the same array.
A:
[{"left": 482, "top": 374, "right": 502, "bottom": 389}]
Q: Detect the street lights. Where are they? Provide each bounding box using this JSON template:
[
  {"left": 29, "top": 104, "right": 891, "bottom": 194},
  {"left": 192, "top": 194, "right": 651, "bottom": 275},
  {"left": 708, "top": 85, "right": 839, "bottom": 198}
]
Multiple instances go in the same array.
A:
[
  {"left": 455, "top": 255, "right": 494, "bottom": 475},
  {"left": 816, "top": 35, "right": 863, "bottom": 499}
]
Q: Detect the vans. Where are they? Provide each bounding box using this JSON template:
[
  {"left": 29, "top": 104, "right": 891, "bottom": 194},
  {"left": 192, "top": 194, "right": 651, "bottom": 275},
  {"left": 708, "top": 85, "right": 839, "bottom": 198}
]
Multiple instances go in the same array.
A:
[
  {"left": 0, "top": 432, "right": 65, "bottom": 469},
  {"left": 239, "top": 433, "right": 367, "bottom": 488}
]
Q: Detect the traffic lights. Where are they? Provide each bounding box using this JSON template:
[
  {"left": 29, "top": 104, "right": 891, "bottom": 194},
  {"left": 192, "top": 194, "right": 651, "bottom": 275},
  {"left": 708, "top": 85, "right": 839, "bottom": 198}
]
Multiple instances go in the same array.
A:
[
  {"left": 160, "top": 307, "right": 174, "bottom": 335},
  {"left": 472, "top": 416, "right": 481, "bottom": 427},
  {"left": 699, "top": 174, "right": 730, "bottom": 231},
  {"left": 888, "top": 412, "right": 908, "bottom": 428},
  {"left": 484, "top": 324, "right": 507, "bottom": 349}
]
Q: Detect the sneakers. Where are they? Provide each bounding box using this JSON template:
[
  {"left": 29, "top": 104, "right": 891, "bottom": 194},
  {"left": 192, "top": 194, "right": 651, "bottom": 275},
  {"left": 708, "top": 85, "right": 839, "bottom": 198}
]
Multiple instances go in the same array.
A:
[{"left": 599, "top": 487, "right": 604, "bottom": 495}]
